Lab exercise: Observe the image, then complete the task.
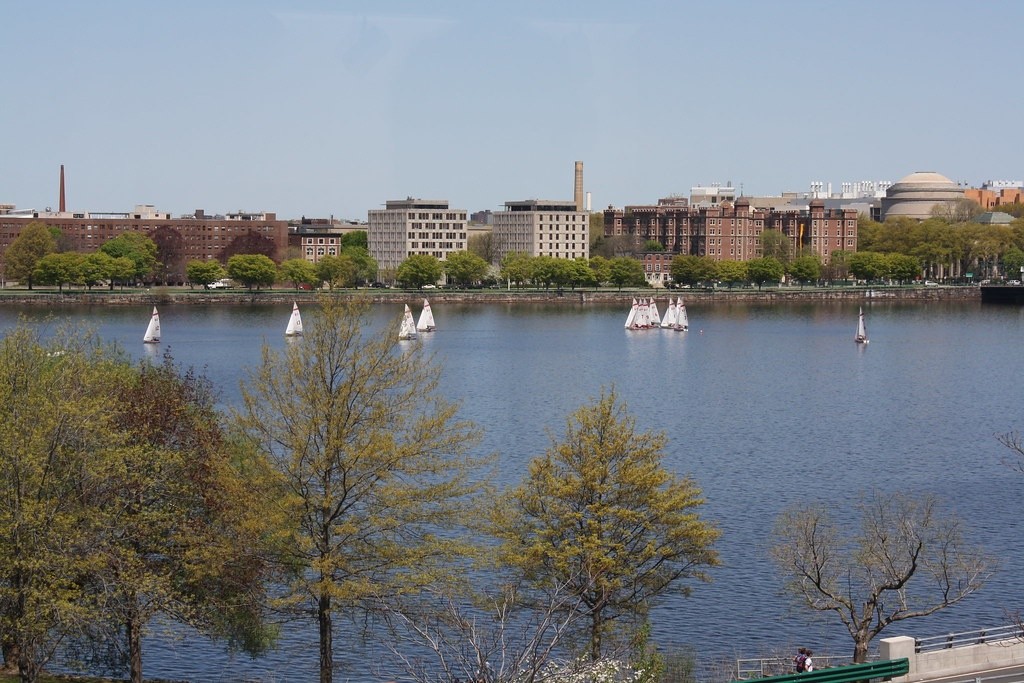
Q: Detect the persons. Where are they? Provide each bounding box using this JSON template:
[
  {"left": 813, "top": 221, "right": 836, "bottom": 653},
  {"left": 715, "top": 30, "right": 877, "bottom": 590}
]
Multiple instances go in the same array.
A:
[{"left": 795, "top": 648, "right": 813, "bottom": 673}]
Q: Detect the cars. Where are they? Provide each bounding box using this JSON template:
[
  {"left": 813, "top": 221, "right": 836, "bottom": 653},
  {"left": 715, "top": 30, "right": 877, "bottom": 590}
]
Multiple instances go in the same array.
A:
[
  {"left": 682, "top": 285, "right": 694, "bottom": 289},
  {"left": 368, "top": 281, "right": 390, "bottom": 289},
  {"left": 420, "top": 284, "right": 450, "bottom": 289},
  {"left": 924, "top": 280, "right": 938, "bottom": 287},
  {"left": 298, "top": 283, "right": 313, "bottom": 290},
  {"left": 489, "top": 284, "right": 502, "bottom": 290},
  {"left": 1007, "top": 280, "right": 1021, "bottom": 286}
]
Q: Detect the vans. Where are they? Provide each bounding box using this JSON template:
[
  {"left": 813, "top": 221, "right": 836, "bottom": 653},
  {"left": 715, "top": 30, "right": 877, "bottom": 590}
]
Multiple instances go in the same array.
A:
[{"left": 208, "top": 278, "right": 235, "bottom": 290}]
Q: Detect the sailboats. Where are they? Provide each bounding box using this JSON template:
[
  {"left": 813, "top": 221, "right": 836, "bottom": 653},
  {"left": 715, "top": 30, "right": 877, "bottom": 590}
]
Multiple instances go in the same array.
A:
[
  {"left": 399, "top": 303, "right": 416, "bottom": 340},
  {"left": 855, "top": 306, "right": 867, "bottom": 342},
  {"left": 416, "top": 299, "right": 437, "bottom": 332},
  {"left": 143, "top": 306, "right": 161, "bottom": 343},
  {"left": 285, "top": 301, "right": 304, "bottom": 337},
  {"left": 625, "top": 296, "right": 689, "bottom": 331}
]
